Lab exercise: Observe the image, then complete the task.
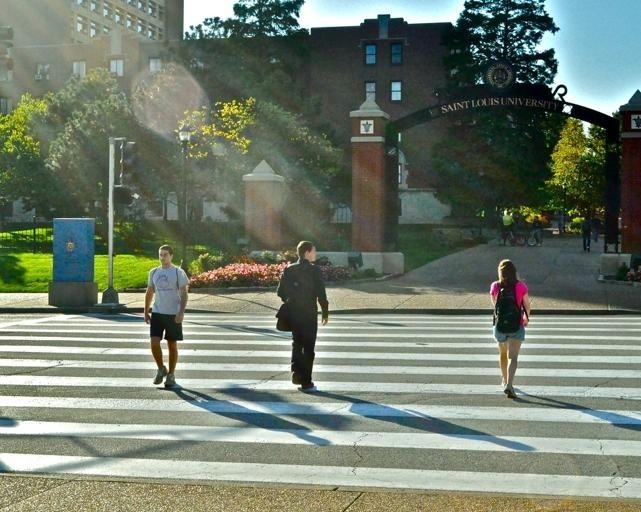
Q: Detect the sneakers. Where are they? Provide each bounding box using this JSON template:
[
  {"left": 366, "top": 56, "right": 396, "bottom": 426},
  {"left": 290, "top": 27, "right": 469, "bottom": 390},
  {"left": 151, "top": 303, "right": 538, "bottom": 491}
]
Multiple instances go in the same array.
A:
[
  {"left": 165, "top": 372, "right": 176, "bottom": 387},
  {"left": 292, "top": 372, "right": 314, "bottom": 389},
  {"left": 153, "top": 366, "right": 167, "bottom": 385},
  {"left": 502, "top": 382, "right": 518, "bottom": 399}
]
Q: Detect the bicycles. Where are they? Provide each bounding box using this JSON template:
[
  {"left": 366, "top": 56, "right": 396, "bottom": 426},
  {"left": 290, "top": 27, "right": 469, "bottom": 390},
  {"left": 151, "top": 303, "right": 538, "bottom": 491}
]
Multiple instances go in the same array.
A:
[{"left": 505, "top": 223, "right": 539, "bottom": 246}]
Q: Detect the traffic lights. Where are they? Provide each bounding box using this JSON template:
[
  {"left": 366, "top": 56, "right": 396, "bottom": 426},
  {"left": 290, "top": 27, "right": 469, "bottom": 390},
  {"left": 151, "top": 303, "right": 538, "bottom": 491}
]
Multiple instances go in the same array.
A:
[
  {"left": 114, "top": 185, "right": 133, "bottom": 206},
  {"left": 120, "top": 140, "right": 138, "bottom": 184}
]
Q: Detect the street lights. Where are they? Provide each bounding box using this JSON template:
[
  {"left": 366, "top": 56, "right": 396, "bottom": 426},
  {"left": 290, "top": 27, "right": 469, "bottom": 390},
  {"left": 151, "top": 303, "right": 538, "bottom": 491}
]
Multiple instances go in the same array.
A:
[{"left": 177, "top": 123, "right": 190, "bottom": 277}]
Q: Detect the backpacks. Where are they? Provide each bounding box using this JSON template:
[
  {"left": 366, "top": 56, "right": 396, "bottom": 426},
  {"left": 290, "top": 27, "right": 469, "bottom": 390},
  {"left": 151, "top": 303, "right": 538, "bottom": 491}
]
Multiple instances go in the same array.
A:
[{"left": 495, "top": 279, "right": 521, "bottom": 334}]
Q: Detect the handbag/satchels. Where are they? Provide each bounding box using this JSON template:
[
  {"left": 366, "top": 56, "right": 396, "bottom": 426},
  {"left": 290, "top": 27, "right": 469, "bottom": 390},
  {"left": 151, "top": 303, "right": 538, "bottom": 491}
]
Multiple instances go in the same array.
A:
[{"left": 275, "top": 303, "right": 291, "bottom": 333}]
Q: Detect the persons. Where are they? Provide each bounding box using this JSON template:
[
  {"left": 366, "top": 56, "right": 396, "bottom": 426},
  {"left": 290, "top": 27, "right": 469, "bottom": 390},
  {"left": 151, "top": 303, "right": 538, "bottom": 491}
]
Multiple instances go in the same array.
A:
[
  {"left": 501, "top": 207, "right": 547, "bottom": 247},
  {"left": 276, "top": 240, "right": 330, "bottom": 390},
  {"left": 592, "top": 214, "right": 602, "bottom": 244},
  {"left": 144, "top": 244, "right": 192, "bottom": 386},
  {"left": 490, "top": 258, "right": 532, "bottom": 398},
  {"left": 581, "top": 213, "right": 592, "bottom": 253}
]
